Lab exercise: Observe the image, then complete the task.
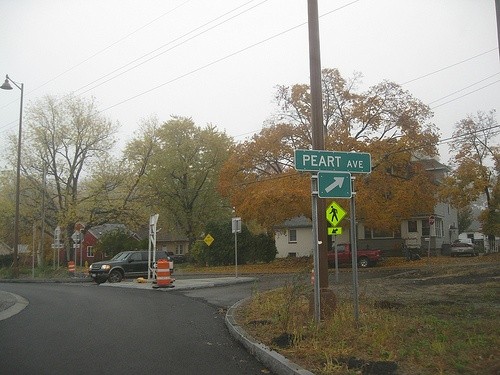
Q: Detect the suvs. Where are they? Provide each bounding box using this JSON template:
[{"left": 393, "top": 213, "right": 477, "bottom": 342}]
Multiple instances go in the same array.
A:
[{"left": 89, "top": 251, "right": 173, "bottom": 284}]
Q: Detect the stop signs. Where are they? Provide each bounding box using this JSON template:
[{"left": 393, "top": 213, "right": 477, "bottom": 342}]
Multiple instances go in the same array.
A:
[{"left": 429, "top": 215, "right": 435, "bottom": 224}]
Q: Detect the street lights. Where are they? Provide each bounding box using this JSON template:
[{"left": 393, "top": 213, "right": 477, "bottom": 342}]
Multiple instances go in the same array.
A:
[{"left": 0, "top": 73, "right": 24, "bottom": 266}]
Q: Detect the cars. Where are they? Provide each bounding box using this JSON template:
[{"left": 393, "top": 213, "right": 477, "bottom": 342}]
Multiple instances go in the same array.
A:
[{"left": 449, "top": 239, "right": 480, "bottom": 258}]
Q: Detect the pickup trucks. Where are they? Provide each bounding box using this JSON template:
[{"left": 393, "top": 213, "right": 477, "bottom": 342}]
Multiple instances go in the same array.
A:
[{"left": 327, "top": 244, "right": 382, "bottom": 267}]
